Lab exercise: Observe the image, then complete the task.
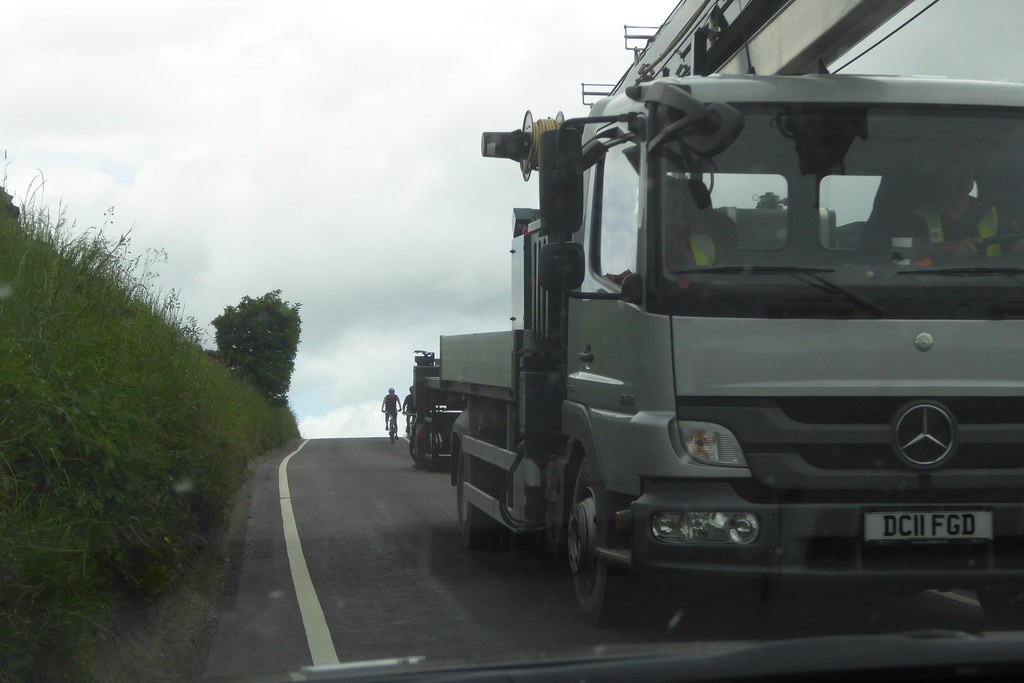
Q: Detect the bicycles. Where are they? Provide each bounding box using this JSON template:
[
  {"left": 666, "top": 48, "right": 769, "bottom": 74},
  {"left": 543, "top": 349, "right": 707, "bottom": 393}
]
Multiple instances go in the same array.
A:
[
  {"left": 381, "top": 408, "right": 402, "bottom": 444},
  {"left": 401, "top": 411, "right": 417, "bottom": 440}
]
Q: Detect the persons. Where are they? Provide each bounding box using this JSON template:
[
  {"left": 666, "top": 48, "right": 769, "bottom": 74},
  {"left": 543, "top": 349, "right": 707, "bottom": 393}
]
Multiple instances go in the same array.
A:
[
  {"left": 403, "top": 385, "right": 417, "bottom": 434},
  {"left": 381, "top": 388, "right": 402, "bottom": 441},
  {"left": 664, "top": 175, "right": 730, "bottom": 267},
  {"left": 900, "top": 161, "right": 1024, "bottom": 258}
]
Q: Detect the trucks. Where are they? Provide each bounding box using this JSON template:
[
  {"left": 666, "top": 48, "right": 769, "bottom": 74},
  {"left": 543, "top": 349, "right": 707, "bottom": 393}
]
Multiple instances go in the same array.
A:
[{"left": 405, "top": 0, "right": 1024, "bottom": 640}]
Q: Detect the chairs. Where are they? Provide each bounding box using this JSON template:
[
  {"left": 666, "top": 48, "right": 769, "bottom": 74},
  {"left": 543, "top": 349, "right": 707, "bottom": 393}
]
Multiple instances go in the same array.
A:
[{"left": 661, "top": 177, "right": 737, "bottom": 268}]
